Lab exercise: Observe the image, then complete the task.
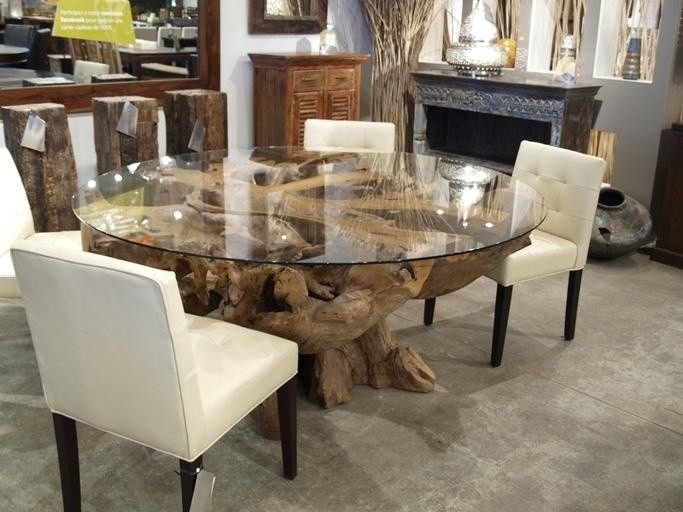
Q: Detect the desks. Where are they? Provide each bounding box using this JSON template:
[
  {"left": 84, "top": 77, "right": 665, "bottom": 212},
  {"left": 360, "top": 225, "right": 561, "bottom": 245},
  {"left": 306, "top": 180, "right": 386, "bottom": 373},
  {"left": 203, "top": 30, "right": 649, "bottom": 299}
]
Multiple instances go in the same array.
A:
[{"left": 70, "top": 151, "right": 551, "bottom": 407}]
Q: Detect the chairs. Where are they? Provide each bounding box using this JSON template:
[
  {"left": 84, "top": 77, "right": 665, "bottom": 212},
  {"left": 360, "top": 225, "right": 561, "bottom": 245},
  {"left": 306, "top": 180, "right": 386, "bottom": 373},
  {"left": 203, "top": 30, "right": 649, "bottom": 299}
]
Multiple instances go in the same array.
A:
[
  {"left": 1, "top": 142, "right": 85, "bottom": 303},
  {"left": 0, "top": 24, "right": 199, "bottom": 88},
  {"left": 9, "top": 241, "right": 300, "bottom": 510},
  {"left": 304, "top": 118, "right": 396, "bottom": 149},
  {"left": 423, "top": 140, "right": 608, "bottom": 368}
]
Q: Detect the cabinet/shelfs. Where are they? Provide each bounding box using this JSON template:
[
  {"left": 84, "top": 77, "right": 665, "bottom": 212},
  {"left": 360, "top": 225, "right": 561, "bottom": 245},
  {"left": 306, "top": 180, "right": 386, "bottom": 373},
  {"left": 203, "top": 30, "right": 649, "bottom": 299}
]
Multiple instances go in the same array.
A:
[{"left": 246, "top": 52, "right": 372, "bottom": 168}]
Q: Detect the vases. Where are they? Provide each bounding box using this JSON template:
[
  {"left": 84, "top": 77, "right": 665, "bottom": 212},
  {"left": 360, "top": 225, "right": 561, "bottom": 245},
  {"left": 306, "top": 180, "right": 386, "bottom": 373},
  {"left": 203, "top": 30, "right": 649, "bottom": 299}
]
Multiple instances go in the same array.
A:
[{"left": 620, "top": 38, "right": 642, "bottom": 81}]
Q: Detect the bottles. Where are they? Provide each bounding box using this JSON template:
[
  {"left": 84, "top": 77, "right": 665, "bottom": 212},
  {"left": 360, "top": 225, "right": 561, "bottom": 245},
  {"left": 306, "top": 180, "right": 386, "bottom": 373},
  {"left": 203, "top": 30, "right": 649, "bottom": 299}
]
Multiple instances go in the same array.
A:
[
  {"left": 555, "top": 31, "right": 578, "bottom": 85},
  {"left": 319, "top": 24, "right": 337, "bottom": 56}
]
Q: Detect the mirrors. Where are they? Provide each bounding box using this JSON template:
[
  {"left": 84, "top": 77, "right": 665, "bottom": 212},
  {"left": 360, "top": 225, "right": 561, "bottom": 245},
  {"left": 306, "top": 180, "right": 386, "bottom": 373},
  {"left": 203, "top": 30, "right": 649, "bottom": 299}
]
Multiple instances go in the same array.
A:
[{"left": 248, "top": 1, "right": 328, "bottom": 36}]
